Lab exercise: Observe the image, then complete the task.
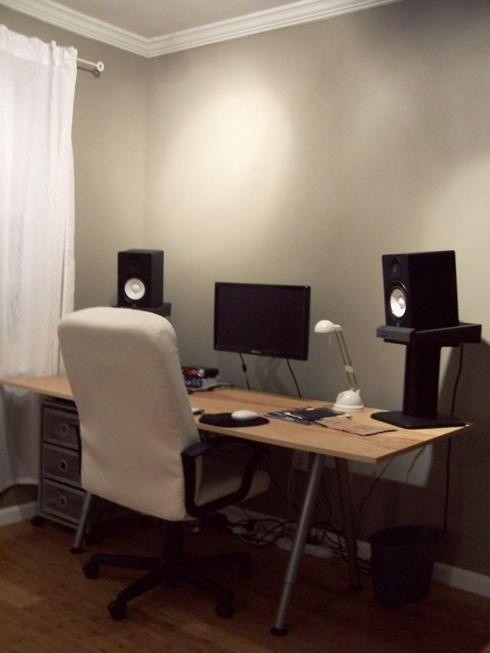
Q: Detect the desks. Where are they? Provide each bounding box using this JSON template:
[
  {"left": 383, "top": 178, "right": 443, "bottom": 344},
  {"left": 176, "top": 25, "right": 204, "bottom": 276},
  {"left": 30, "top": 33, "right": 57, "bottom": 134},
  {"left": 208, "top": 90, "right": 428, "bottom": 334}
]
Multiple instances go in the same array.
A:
[{"left": 1, "top": 375, "right": 477, "bottom": 637}]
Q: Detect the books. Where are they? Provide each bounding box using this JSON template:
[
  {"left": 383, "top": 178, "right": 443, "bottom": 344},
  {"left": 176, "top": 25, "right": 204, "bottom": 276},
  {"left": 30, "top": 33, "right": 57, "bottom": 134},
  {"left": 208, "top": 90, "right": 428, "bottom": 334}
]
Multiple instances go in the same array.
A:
[
  {"left": 183, "top": 362, "right": 222, "bottom": 392},
  {"left": 267, "top": 405, "right": 351, "bottom": 423}
]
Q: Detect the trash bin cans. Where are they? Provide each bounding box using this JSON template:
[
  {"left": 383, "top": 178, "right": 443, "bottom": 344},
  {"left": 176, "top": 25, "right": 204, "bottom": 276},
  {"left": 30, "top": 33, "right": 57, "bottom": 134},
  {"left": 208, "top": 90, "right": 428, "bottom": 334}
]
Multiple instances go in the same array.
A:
[{"left": 365, "top": 525, "right": 436, "bottom": 607}]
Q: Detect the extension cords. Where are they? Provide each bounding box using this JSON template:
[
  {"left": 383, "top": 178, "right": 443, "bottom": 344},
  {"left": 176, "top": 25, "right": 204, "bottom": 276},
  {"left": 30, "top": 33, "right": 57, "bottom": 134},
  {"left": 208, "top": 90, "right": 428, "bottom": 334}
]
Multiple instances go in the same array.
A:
[{"left": 277, "top": 538, "right": 333, "bottom": 558}]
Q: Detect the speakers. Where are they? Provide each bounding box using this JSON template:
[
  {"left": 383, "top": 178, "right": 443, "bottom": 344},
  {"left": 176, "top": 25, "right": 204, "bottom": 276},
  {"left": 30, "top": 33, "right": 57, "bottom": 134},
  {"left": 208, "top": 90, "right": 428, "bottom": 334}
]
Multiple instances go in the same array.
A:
[
  {"left": 382, "top": 250, "right": 458, "bottom": 331},
  {"left": 117, "top": 248, "right": 163, "bottom": 308}
]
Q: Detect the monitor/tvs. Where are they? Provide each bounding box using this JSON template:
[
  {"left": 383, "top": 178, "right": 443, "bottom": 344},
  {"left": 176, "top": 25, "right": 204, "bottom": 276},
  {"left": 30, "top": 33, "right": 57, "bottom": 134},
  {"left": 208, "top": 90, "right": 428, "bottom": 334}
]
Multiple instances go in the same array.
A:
[{"left": 214, "top": 282, "right": 310, "bottom": 360}]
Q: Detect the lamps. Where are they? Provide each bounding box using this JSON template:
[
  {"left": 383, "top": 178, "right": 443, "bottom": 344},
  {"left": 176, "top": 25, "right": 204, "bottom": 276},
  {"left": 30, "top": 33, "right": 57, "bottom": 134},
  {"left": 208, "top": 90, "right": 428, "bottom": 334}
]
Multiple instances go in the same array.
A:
[{"left": 314, "top": 319, "right": 365, "bottom": 414}]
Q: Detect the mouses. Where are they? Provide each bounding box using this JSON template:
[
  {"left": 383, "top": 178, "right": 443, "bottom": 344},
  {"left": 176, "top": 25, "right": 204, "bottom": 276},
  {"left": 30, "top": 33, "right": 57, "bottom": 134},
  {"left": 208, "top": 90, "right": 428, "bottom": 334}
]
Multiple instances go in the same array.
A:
[{"left": 231, "top": 409, "right": 259, "bottom": 421}]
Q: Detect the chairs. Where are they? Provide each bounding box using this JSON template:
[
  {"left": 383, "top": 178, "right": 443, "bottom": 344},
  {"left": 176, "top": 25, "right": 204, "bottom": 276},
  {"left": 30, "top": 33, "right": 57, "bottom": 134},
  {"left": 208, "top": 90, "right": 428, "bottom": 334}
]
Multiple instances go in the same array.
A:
[{"left": 57, "top": 306, "right": 270, "bottom": 621}]
